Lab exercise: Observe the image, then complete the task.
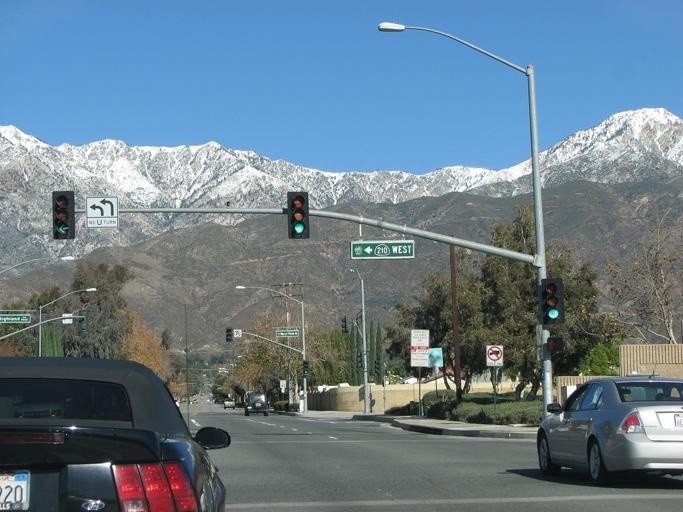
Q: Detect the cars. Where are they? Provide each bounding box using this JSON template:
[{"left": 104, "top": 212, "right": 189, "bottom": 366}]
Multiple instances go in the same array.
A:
[
  {"left": 168, "top": 381, "right": 218, "bottom": 408},
  {"left": 534, "top": 357, "right": 683, "bottom": 484},
  {"left": 0, "top": 353, "right": 233, "bottom": 512},
  {"left": 222, "top": 396, "right": 236, "bottom": 410}
]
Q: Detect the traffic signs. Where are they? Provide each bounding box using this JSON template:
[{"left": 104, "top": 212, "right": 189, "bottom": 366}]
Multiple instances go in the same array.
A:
[
  {"left": 0, "top": 314, "right": 31, "bottom": 324},
  {"left": 349, "top": 240, "right": 416, "bottom": 259},
  {"left": 274, "top": 329, "right": 300, "bottom": 338}
]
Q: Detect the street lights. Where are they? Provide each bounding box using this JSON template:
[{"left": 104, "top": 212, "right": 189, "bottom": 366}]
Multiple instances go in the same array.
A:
[
  {"left": 349, "top": 266, "right": 372, "bottom": 415},
  {"left": 374, "top": 21, "right": 560, "bottom": 422},
  {"left": 0, "top": 310, "right": 87, "bottom": 339},
  {"left": 234, "top": 284, "right": 308, "bottom": 412},
  {"left": 37, "top": 287, "right": 97, "bottom": 356}
]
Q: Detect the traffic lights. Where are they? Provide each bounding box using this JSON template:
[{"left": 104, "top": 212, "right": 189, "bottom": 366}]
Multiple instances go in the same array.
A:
[
  {"left": 285, "top": 191, "right": 311, "bottom": 239},
  {"left": 302, "top": 361, "right": 308, "bottom": 377},
  {"left": 341, "top": 313, "right": 350, "bottom": 333},
  {"left": 224, "top": 326, "right": 233, "bottom": 344},
  {"left": 50, "top": 192, "right": 75, "bottom": 240},
  {"left": 541, "top": 277, "right": 565, "bottom": 325}
]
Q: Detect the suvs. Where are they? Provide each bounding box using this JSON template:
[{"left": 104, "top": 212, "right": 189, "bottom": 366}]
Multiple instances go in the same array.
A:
[{"left": 243, "top": 391, "right": 271, "bottom": 418}]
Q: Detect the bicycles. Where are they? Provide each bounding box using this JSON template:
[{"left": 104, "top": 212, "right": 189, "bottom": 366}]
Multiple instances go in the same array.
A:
[{"left": 0, "top": 254, "right": 76, "bottom": 274}]
